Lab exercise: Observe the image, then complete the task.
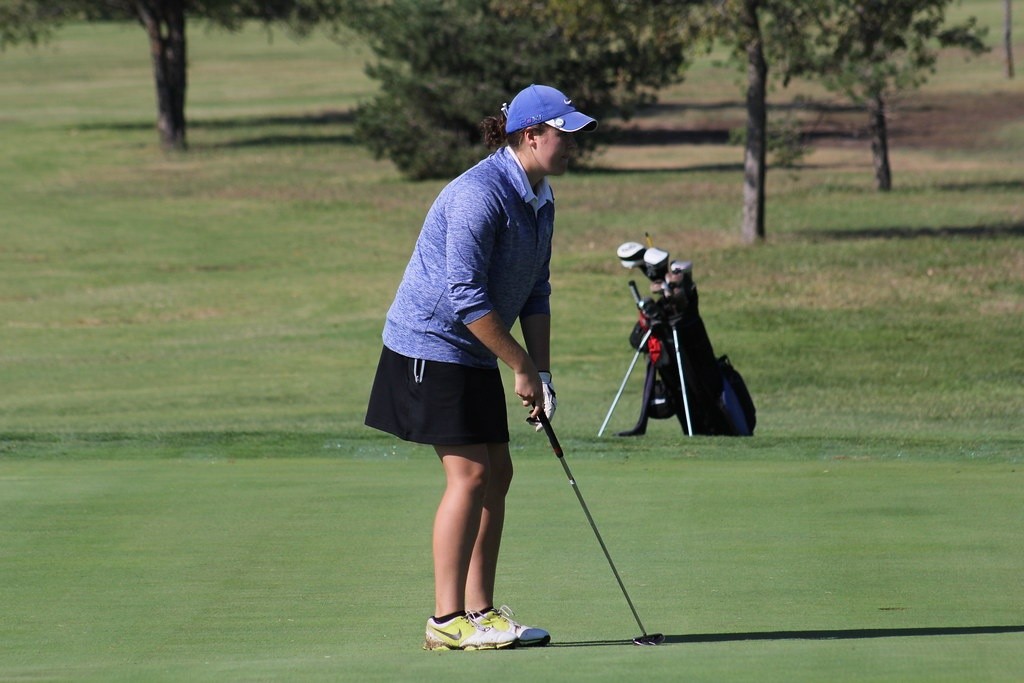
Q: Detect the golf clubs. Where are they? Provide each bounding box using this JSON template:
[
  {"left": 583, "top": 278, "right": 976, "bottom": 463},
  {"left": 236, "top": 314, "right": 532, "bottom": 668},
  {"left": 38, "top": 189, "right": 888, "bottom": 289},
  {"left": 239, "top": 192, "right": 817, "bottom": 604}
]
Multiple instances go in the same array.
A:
[
  {"left": 532, "top": 400, "right": 665, "bottom": 648},
  {"left": 613, "top": 240, "right": 694, "bottom": 321}
]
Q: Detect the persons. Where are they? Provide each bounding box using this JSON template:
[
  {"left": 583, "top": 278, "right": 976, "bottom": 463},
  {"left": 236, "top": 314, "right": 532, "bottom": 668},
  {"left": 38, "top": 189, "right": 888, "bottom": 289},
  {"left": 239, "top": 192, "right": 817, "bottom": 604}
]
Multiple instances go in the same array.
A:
[{"left": 362, "top": 81, "right": 601, "bottom": 652}]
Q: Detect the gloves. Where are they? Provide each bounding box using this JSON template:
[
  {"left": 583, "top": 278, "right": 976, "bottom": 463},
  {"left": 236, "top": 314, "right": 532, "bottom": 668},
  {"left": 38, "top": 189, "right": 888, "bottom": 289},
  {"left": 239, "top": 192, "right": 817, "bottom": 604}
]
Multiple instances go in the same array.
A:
[{"left": 527, "top": 371, "right": 557, "bottom": 432}]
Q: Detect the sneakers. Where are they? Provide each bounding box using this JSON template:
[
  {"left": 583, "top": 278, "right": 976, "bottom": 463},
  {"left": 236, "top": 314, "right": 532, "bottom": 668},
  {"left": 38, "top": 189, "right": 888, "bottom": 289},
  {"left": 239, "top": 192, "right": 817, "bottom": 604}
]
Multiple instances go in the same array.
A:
[
  {"left": 468, "top": 608, "right": 550, "bottom": 646},
  {"left": 421, "top": 617, "right": 518, "bottom": 651}
]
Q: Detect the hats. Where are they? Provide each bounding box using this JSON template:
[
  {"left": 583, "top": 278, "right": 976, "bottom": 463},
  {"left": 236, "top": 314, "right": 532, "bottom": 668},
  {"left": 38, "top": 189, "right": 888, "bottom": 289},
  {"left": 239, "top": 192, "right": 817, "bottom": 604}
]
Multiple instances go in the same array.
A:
[{"left": 504, "top": 84, "right": 598, "bottom": 136}]
